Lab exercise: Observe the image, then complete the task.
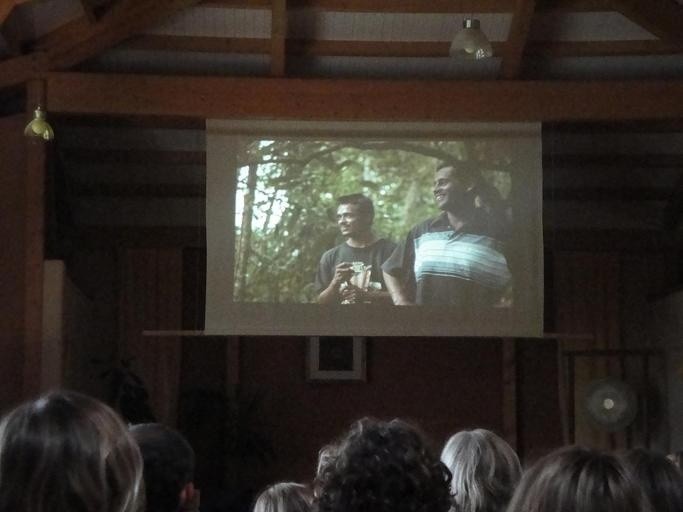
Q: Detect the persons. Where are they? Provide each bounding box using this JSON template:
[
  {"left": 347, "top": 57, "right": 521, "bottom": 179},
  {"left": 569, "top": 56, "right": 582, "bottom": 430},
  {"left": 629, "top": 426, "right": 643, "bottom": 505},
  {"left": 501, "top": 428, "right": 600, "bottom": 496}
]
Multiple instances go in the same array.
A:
[
  {"left": 473, "top": 186, "right": 515, "bottom": 245},
  {"left": 620, "top": 449, "right": 683, "bottom": 512},
  {"left": 313, "top": 417, "right": 452, "bottom": 512},
  {"left": 252, "top": 483, "right": 317, "bottom": 511},
  {"left": 508, "top": 447, "right": 655, "bottom": 512},
  {"left": 382, "top": 160, "right": 517, "bottom": 308},
  {"left": 443, "top": 427, "right": 524, "bottom": 510},
  {"left": 313, "top": 194, "right": 401, "bottom": 306},
  {"left": 129, "top": 424, "right": 197, "bottom": 512},
  {"left": 0, "top": 392, "right": 143, "bottom": 509}
]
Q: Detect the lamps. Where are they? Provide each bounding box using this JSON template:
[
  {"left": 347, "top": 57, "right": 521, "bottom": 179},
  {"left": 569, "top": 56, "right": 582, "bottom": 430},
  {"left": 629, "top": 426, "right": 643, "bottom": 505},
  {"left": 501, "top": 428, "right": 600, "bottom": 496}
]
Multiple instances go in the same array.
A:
[
  {"left": 442, "top": 11, "right": 496, "bottom": 62},
  {"left": 17, "top": 101, "right": 57, "bottom": 142}
]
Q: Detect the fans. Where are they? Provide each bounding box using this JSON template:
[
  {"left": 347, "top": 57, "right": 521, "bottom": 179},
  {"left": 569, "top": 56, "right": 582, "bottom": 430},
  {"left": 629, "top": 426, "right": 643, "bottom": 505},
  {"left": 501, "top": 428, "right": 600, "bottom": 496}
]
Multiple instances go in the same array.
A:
[{"left": 580, "top": 378, "right": 641, "bottom": 429}]
